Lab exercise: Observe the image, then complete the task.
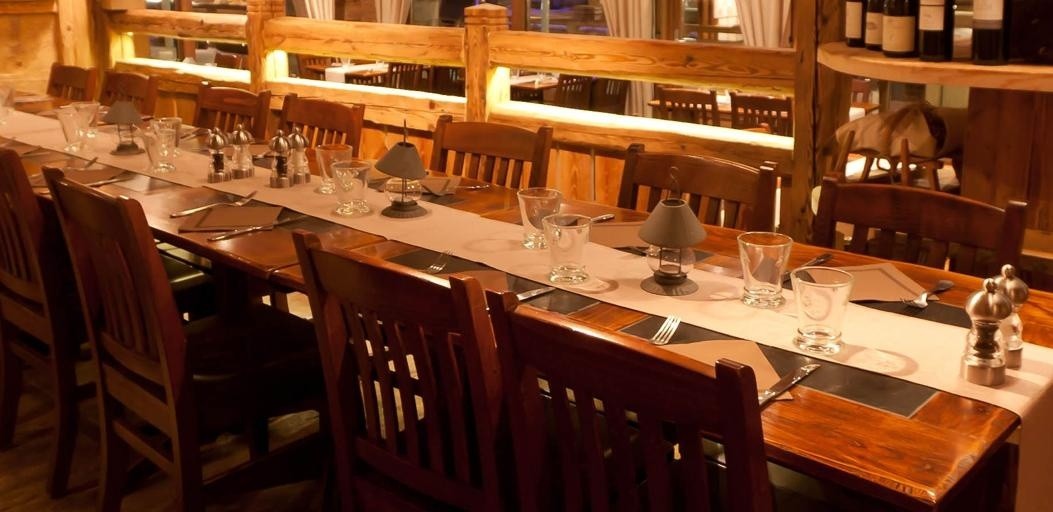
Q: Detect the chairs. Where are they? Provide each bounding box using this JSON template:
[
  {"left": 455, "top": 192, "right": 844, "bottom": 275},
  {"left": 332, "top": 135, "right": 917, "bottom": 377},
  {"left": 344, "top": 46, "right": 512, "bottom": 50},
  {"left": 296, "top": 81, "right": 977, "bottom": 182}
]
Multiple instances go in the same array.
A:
[{"left": 47, "top": 53, "right": 879, "bottom": 144}]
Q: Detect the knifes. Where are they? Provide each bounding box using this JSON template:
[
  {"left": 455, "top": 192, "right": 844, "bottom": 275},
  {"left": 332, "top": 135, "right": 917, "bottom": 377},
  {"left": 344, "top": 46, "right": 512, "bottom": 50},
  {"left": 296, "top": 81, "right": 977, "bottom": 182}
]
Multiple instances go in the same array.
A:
[
  {"left": 514, "top": 286, "right": 557, "bottom": 301},
  {"left": 210, "top": 210, "right": 311, "bottom": 245},
  {"left": 39, "top": 173, "right": 133, "bottom": 193},
  {"left": 756, "top": 363, "right": 822, "bottom": 407},
  {"left": 776, "top": 250, "right": 831, "bottom": 283}
]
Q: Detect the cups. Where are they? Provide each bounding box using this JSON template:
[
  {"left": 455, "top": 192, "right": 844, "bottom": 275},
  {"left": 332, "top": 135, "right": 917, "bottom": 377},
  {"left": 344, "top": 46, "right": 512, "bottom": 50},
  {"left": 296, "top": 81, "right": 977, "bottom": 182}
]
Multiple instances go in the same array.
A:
[
  {"left": 540, "top": 213, "right": 593, "bottom": 286},
  {"left": 736, "top": 229, "right": 794, "bottom": 307},
  {"left": 56, "top": 98, "right": 101, "bottom": 154},
  {"left": 139, "top": 116, "right": 186, "bottom": 176},
  {"left": 516, "top": 187, "right": 562, "bottom": 249},
  {"left": 333, "top": 58, "right": 385, "bottom": 71},
  {"left": 315, "top": 143, "right": 371, "bottom": 218},
  {"left": 513, "top": 69, "right": 558, "bottom": 84},
  {"left": 791, "top": 267, "right": 854, "bottom": 352}
]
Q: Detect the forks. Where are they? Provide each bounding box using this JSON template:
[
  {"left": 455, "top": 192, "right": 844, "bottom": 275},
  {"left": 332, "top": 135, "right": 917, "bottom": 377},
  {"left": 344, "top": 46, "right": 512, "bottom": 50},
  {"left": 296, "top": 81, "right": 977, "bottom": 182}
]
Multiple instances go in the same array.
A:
[
  {"left": 32, "top": 156, "right": 103, "bottom": 179},
  {"left": 423, "top": 248, "right": 455, "bottom": 272},
  {"left": 902, "top": 278, "right": 956, "bottom": 308},
  {"left": 170, "top": 189, "right": 260, "bottom": 220},
  {"left": 650, "top": 316, "right": 679, "bottom": 345},
  {"left": 421, "top": 183, "right": 490, "bottom": 197}
]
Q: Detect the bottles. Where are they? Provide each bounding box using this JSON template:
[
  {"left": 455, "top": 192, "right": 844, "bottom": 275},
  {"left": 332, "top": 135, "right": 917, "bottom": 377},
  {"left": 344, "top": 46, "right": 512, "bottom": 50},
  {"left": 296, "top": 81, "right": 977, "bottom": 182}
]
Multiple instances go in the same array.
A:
[
  {"left": 208, "top": 122, "right": 311, "bottom": 190},
  {"left": 839, "top": 1, "right": 1007, "bottom": 67},
  {"left": 961, "top": 262, "right": 1033, "bottom": 388}
]
